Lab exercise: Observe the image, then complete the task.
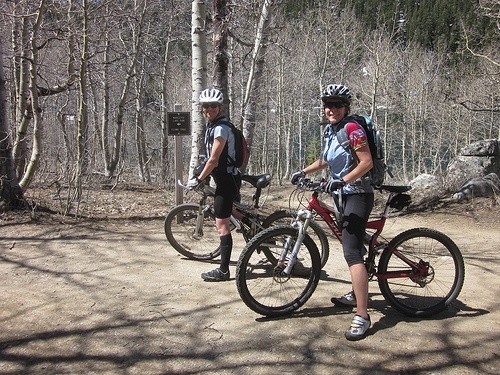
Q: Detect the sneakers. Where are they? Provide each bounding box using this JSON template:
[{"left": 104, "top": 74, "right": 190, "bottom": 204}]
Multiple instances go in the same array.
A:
[
  {"left": 201, "top": 268, "right": 230, "bottom": 281},
  {"left": 345, "top": 313, "right": 371, "bottom": 340},
  {"left": 331, "top": 291, "right": 358, "bottom": 307}
]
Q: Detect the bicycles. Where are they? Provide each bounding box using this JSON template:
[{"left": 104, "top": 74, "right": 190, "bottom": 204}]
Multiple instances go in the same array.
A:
[
  {"left": 235, "top": 175, "right": 465, "bottom": 319},
  {"left": 164, "top": 157, "right": 329, "bottom": 278}
]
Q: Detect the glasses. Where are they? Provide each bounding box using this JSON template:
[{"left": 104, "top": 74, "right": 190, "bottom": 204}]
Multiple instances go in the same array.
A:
[
  {"left": 324, "top": 101, "right": 345, "bottom": 108},
  {"left": 202, "top": 104, "right": 218, "bottom": 108}
]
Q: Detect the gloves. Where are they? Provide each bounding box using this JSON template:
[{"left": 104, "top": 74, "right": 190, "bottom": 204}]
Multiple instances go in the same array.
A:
[
  {"left": 325, "top": 176, "right": 346, "bottom": 196},
  {"left": 290, "top": 171, "right": 305, "bottom": 185},
  {"left": 187, "top": 177, "right": 200, "bottom": 189}
]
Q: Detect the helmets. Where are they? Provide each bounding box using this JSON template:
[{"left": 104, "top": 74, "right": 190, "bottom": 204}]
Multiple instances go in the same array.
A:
[
  {"left": 199, "top": 88, "right": 223, "bottom": 104},
  {"left": 321, "top": 84, "right": 351, "bottom": 104}
]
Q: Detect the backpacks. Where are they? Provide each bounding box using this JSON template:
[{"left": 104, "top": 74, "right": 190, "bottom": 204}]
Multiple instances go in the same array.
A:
[
  {"left": 337, "top": 114, "right": 386, "bottom": 186},
  {"left": 212, "top": 121, "right": 247, "bottom": 167}
]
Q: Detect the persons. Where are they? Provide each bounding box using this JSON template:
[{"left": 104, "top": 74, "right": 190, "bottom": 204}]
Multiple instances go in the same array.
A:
[
  {"left": 291, "top": 84, "right": 374, "bottom": 341},
  {"left": 186, "top": 89, "right": 241, "bottom": 281}
]
